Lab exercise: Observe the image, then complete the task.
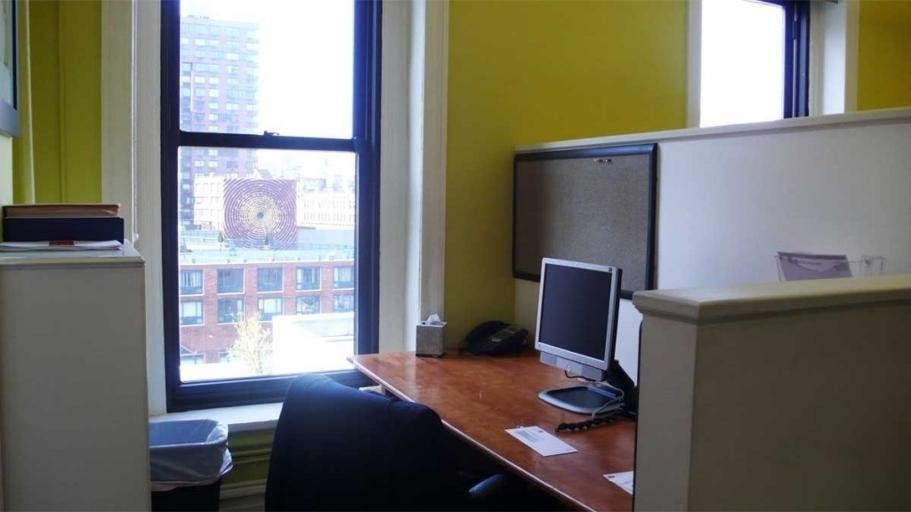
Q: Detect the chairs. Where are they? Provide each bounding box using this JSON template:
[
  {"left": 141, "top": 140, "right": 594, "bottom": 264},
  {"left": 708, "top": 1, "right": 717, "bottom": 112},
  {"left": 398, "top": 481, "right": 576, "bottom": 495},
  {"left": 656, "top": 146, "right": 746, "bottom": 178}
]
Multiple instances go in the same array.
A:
[{"left": 264, "top": 367, "right": 514, "bottom": 509}]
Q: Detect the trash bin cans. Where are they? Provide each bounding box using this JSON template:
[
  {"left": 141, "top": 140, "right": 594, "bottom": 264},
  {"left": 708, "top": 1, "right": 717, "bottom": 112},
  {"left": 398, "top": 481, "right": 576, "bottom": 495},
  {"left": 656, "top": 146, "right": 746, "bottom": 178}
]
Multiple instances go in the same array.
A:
[{"left": 150, "top": 417, "right": 234, "bottom": 512}]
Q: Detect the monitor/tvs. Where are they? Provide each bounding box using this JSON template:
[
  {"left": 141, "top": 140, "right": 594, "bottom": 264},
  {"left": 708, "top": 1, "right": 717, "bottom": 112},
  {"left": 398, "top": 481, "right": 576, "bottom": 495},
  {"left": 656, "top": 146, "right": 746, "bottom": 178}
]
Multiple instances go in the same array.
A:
[{"left": 534, "top": 257, "right": 635, "bottom": 415}]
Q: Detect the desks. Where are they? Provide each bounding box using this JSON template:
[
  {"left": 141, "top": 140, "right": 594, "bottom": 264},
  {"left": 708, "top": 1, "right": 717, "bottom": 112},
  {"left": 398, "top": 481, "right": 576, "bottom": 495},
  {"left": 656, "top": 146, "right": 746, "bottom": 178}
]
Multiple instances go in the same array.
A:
[{"left": 347, "top": 347, "right": 634, "bottom": 512}]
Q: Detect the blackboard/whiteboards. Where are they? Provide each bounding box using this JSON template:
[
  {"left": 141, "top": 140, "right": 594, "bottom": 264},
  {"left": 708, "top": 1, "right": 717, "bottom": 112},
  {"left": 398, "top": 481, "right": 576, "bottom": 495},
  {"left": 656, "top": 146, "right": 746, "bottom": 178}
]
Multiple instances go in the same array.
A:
[{"left": 466, "top": 321, "right": 530, "bottom": 357}]
